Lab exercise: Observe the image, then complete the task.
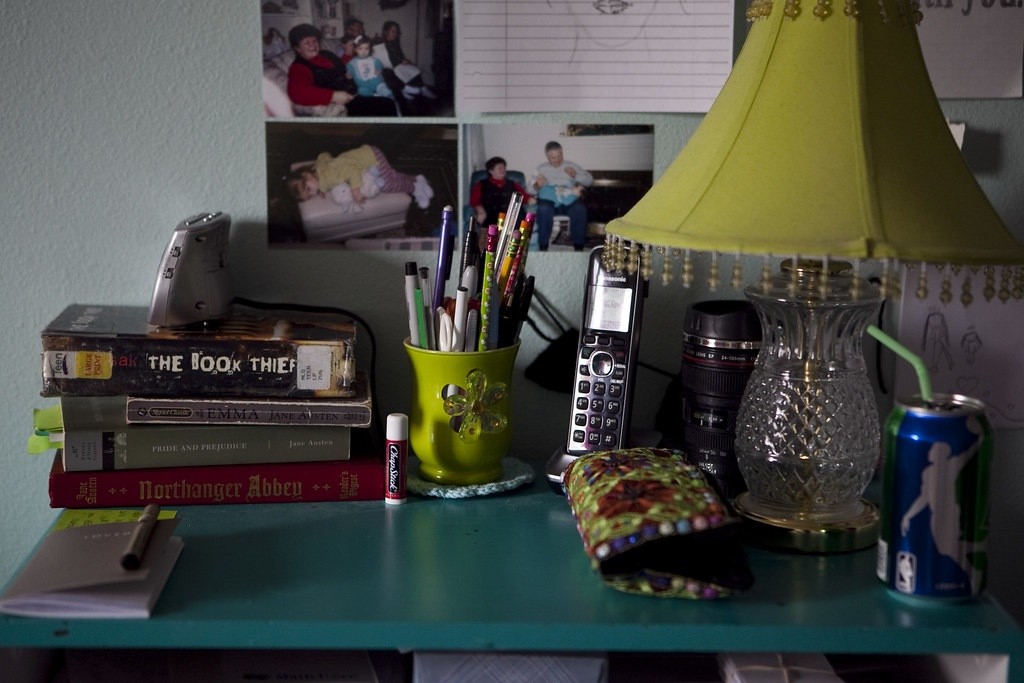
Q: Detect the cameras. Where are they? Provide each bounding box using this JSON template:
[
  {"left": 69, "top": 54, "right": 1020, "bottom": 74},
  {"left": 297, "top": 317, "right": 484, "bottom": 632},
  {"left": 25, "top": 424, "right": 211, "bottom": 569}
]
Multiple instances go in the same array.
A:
[{"left": 657, "top": 297, "right": 765, "bottom": 496}]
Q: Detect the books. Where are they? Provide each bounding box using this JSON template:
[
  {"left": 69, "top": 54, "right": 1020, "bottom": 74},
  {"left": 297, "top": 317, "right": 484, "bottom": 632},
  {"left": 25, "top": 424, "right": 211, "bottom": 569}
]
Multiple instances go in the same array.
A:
[
  {"left": 6, "top": 510, "right": 184, "bottom": 619},
  {"left": 28, "top": 303, "right": 384, "bottom": 508}
]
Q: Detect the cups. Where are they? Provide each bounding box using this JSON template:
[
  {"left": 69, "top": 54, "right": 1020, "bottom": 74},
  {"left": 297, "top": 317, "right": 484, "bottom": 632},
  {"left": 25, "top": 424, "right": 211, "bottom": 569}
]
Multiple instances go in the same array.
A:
[{"left": 405, "top": 334, "right": 521, "bottom": 486}]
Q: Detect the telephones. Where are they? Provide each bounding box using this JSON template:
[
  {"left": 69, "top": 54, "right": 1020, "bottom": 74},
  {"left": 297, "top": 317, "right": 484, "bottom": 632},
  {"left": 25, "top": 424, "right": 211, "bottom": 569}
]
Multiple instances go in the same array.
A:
[{"left": 547, "top": 245, "right": 649, "bottom": 499}]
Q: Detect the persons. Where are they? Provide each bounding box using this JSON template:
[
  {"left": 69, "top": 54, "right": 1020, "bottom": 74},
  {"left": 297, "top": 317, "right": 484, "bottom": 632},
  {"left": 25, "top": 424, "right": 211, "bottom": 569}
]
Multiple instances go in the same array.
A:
[
  {"left": 524, "top": 141, "right": 594, "bottom": 252},
  {"left": 288, "top": 144, "right": 434, "bottom": 211},
  {"left": 469, "top": 156, "right": 537, "bottom": 251},
  {"left": 287, "top": 18, "right": 436, "bottom": 117}
]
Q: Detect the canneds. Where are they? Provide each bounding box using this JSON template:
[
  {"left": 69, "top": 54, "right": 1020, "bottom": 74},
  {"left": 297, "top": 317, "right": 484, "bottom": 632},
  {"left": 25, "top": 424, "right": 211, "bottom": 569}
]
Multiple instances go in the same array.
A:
[{"left": 876, "top": 392, "right": 993, "bottom": 604}]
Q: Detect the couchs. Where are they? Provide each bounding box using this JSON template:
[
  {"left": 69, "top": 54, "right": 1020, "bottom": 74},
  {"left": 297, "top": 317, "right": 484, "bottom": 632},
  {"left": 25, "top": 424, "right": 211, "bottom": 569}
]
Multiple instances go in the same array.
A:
[
  {"left": 263, "top": 38, "right": 346, "bottom": 118},
  {"left": 470, "top": 170, "right": 538, "bottom": 237}
]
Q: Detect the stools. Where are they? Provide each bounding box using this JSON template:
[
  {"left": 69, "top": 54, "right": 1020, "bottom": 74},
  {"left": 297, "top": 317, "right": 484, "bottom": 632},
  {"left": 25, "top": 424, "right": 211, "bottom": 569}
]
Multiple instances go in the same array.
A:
[
  {"left": 553, "top": 215, "right": 572, "bottom": 240},
  {"left": 292, "top": 161, "right": 412, "bottom": 243}
]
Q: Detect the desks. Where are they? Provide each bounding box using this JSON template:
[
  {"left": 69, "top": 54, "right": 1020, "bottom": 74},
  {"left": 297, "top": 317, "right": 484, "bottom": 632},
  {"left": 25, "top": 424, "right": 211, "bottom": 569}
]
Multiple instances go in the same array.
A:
[{"left": 0, "top": 439, "right": 1024, "bottom": 683}]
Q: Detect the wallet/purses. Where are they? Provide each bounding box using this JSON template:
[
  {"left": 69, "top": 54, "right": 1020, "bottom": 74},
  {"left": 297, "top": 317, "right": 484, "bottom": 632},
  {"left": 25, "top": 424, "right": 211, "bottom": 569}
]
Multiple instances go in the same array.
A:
[{"left": 562, "top": 445, "right": 753, "bottom": 602}]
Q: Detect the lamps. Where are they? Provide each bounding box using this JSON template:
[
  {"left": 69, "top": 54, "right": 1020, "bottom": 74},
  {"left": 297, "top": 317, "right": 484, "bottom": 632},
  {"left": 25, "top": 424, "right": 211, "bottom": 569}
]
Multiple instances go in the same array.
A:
[{"left": 605, "top": 0, "right": 1024, "bottom": 556}]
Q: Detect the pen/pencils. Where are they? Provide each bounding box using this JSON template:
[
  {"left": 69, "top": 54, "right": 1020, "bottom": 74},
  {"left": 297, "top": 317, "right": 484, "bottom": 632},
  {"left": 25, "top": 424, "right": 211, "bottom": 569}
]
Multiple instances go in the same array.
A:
[
  {"left": 404, "top": 192, "right": 538, "bottom": 356},
  {"left": 117, "top": 502, "right": 162, "bottom": 569}
]
